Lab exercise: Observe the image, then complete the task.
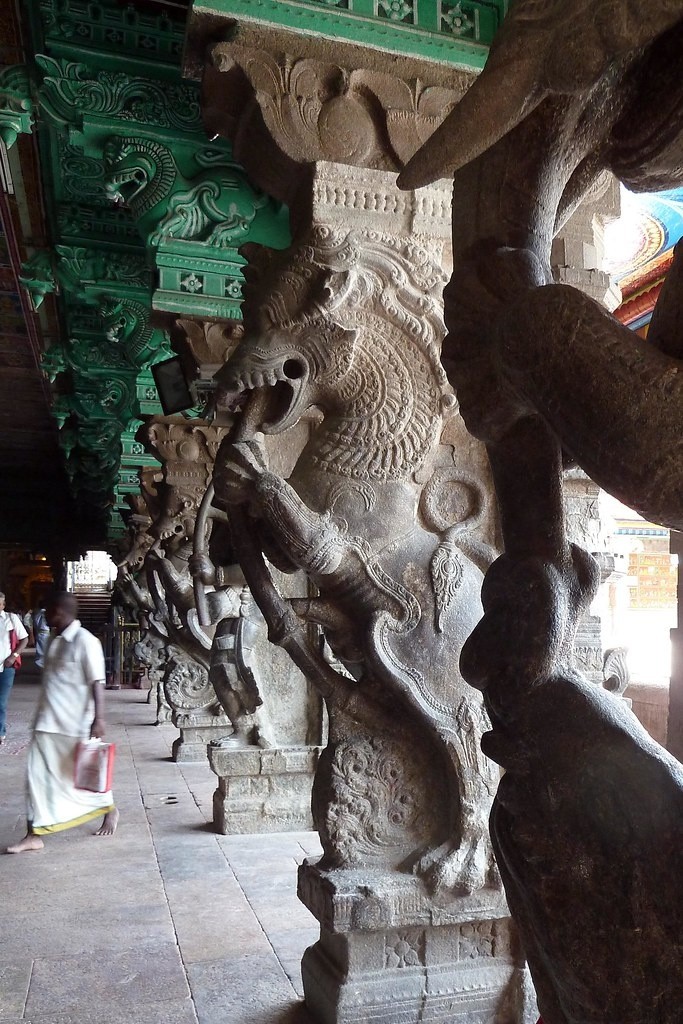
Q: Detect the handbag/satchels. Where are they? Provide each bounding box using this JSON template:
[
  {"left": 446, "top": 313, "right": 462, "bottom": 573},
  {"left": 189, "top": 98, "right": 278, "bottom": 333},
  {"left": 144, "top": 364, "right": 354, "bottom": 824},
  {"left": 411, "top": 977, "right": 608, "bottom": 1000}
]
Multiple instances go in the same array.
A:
[
  {"left": 9, "top": 613, "right": 21, "bottom": 668},
  {"left": 73, "top": 735, "right": 114, "bottom": 792}
]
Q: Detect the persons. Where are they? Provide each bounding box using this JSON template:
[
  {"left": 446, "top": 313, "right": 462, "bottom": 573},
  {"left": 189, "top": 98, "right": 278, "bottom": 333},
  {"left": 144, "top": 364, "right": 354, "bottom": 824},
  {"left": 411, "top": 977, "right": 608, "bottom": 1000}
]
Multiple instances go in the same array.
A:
[
  {"left": 16, "top": 608, "right": 23, "bottom": 624},
  {"left": 23, "top": 609, "right": 33, "bottom": 647},
  {"left": 0, "top": 591, "right": 30, "bottom": 745},
  {"left": 6, "top": 592, "right": 120, "bottom": 854},
  {"left": 33, "top": 601, "right": 52, "bottom": 670}
]
